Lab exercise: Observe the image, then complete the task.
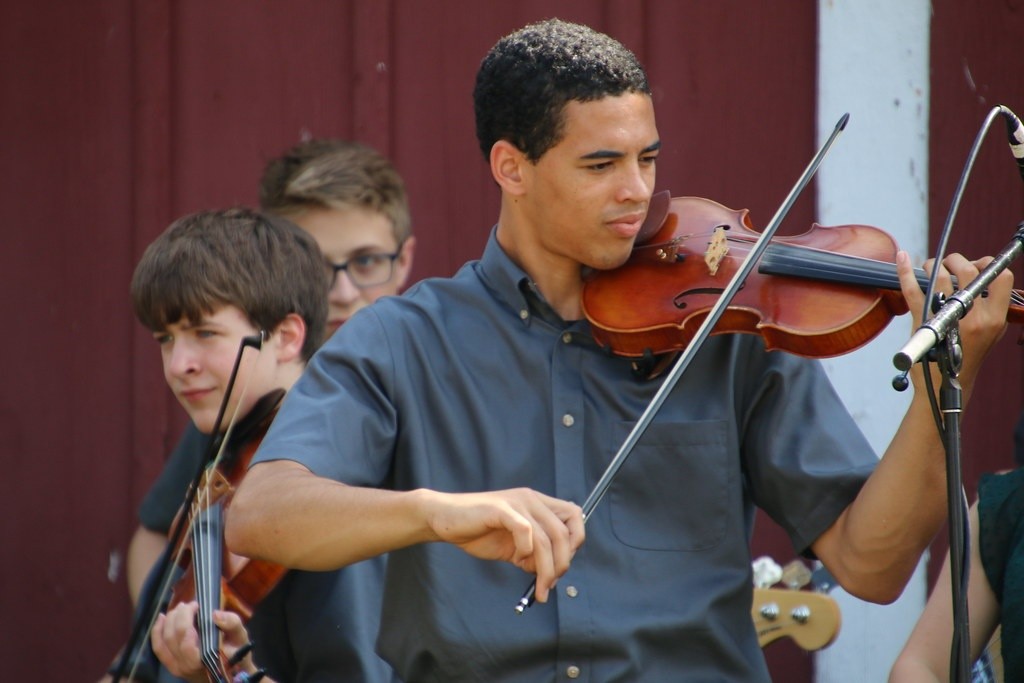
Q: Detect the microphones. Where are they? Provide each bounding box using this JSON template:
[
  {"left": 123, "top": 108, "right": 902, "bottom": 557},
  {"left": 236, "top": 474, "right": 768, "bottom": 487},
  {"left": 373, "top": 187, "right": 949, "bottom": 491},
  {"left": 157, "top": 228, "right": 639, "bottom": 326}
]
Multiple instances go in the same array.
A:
[{"left": 999, "top": 106, "right": 1024, "bottom": 180}]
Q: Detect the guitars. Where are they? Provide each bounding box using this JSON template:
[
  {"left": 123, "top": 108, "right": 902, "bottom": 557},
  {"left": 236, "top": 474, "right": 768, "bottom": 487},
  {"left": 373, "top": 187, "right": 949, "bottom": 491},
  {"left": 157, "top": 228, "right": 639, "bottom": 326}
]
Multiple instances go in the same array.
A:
[{"left": 746, "top": 548, "right": 843, "bottom": 653}]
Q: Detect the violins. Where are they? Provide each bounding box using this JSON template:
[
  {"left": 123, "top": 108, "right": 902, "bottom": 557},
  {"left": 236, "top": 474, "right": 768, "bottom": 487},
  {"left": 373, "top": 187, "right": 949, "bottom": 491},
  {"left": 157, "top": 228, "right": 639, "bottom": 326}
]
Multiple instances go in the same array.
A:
[
  {"left": 168, "top": 387, "right": 295, "bottom": 682},
  {"left": 580, "top": 192, "right": 1024, "bottom": 359}
]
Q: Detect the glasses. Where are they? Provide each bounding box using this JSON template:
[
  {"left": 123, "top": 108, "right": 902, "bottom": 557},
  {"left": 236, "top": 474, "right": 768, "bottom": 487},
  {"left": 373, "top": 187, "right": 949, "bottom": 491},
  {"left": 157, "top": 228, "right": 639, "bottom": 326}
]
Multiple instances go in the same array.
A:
[{"left": 317, "top": 242, "right": 403, "bottom": 295}]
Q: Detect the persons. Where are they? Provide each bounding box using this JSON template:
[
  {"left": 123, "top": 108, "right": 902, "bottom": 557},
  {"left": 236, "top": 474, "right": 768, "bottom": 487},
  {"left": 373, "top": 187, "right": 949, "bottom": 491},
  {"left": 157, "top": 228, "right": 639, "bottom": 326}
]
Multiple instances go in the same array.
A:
[{"left": 127, "top": 17, "right": 1024, "bottom": 683}]
